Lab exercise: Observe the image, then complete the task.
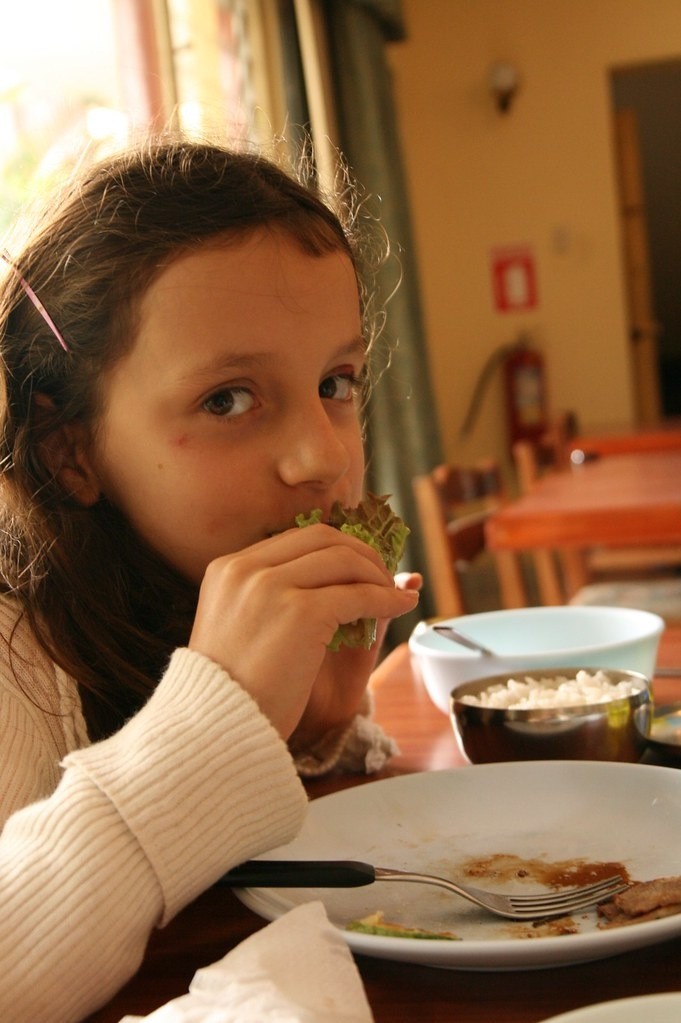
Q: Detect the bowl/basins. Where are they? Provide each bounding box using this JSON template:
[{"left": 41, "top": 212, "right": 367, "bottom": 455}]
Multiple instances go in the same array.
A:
[
  {"left": 409, "top": 607, "right": 664, "bottom": 764},
  {"left": 451, "top": 668, "right": 654, "bottom": 763}
]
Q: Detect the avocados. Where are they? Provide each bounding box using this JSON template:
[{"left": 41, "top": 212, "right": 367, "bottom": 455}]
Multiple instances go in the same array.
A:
[{"left": 347, "top": 909, "right": 462, "bottom": 940}]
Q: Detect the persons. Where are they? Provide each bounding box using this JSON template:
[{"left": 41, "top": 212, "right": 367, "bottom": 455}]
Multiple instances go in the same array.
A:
[{"left": 0, "top": 142, "right": 421, "bottom": 1023}]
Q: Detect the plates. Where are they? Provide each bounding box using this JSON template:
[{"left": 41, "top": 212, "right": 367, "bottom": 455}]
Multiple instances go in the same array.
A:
[{"left": 228, "top": 765, "right": 681, "bottom": 973}]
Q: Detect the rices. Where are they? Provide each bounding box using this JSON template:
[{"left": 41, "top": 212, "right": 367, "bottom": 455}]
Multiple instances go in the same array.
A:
[{"left": 456, "top": 670, "right": 642, "bottom": 710}]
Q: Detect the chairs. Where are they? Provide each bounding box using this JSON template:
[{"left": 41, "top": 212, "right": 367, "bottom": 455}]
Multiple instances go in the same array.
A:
[{"left": 413, "top": 412, "right": 580, "bottom": 621}]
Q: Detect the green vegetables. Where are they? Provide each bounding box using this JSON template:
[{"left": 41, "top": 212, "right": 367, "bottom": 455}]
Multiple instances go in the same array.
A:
[{"left": 266, "top": 491, "right": 411, "bottom": 648}]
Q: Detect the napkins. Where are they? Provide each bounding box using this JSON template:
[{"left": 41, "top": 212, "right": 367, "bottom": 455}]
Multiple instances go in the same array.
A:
[{"left": 119, "top": 902, "right": 375, "bottom": 1022}]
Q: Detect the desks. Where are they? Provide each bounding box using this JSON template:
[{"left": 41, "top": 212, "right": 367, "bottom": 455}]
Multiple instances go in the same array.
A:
[
  {"left": 77, "top": 631, "right": 681, "bottom": 1023},
  {"left": 486, "top": 451, "right": 681, "bottom": 609}
]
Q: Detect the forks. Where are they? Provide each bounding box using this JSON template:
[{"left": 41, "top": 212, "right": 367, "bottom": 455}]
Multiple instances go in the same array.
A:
[{"left": 219, "top": 857, "right": 626, "bottom": 918}]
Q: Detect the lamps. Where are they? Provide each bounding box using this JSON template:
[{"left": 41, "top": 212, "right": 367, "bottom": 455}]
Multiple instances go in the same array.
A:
[{"left": 487, "top": 55, "right": 520, "bottom": 111}]
